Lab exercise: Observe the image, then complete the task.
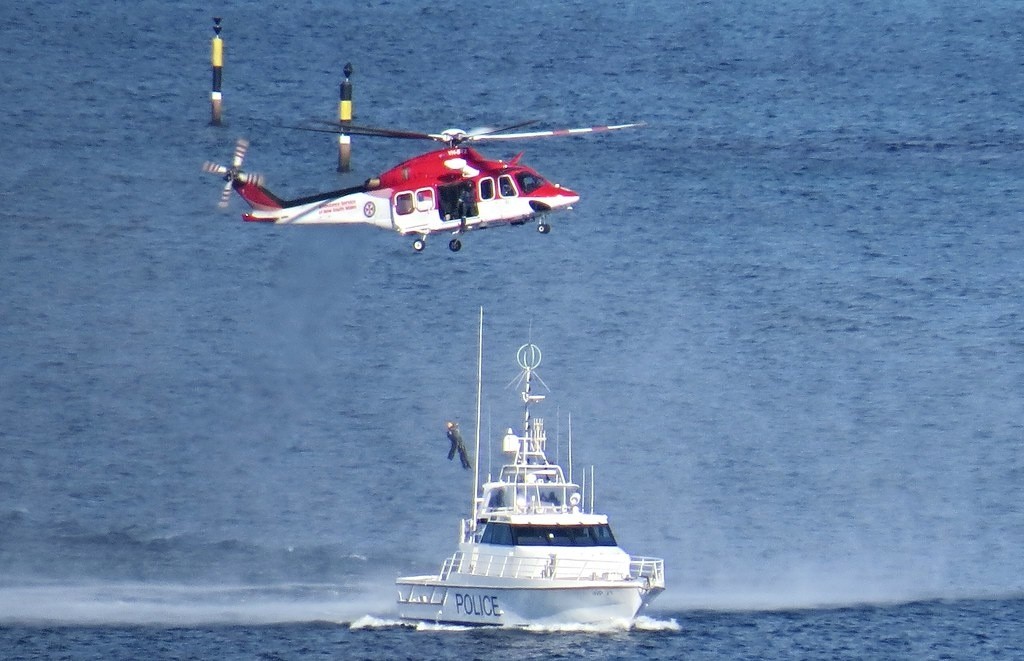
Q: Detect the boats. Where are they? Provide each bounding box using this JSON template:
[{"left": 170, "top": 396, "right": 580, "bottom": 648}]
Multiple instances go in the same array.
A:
[{"left": 392, "top": 307, "right": 668, "bottom": 636}]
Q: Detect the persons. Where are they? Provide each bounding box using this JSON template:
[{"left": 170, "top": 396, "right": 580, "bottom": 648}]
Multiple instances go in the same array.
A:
[
  {"left": 490, "top": 490, "right": 561, "bottom": 507},
  {"left": 447, "top": 422, "right": 471, "bottom": 470},
  {"left": 454, "top": 181, "right": 474, "bottom": 227}
]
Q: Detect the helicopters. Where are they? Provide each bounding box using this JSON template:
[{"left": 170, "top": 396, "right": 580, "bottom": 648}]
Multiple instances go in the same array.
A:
[{"left": 198, "top": 13, "right": 650, "bottom": 254}]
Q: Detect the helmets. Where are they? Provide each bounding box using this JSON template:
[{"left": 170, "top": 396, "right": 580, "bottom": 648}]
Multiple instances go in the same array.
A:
[{"left": 447, "top": 423, "right": 453, "bottom": 428}]
